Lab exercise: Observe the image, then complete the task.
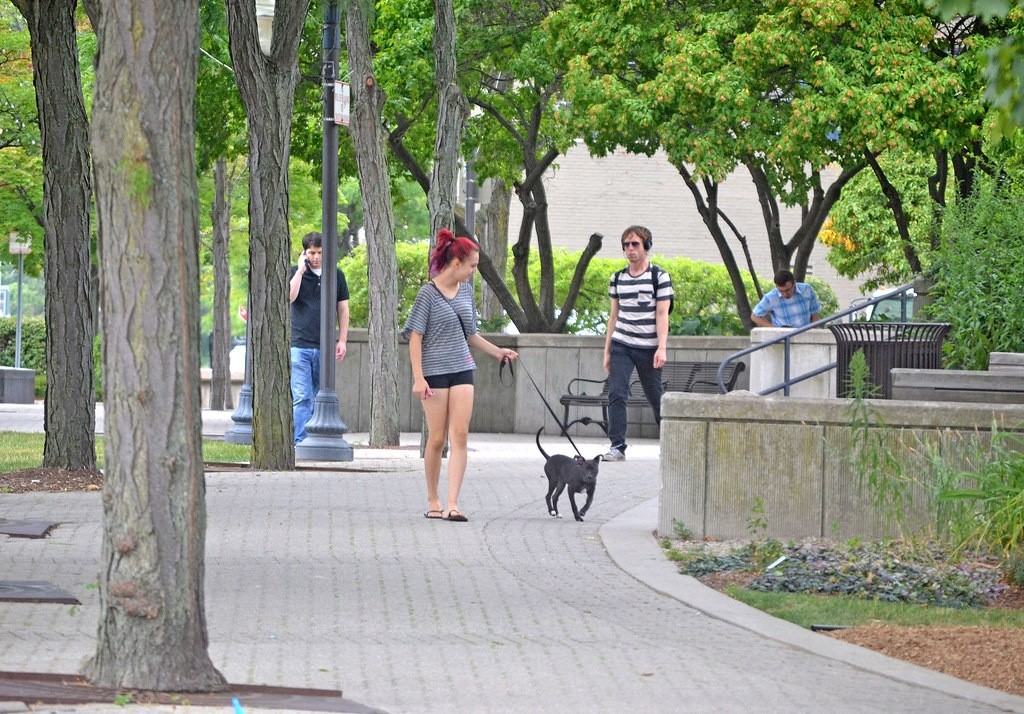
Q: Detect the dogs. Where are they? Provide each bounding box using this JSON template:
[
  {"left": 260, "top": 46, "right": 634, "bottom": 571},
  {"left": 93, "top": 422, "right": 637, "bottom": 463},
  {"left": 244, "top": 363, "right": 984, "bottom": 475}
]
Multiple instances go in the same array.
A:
[{"left": 536, "top": 426, "right": 604, "bottom": 520}]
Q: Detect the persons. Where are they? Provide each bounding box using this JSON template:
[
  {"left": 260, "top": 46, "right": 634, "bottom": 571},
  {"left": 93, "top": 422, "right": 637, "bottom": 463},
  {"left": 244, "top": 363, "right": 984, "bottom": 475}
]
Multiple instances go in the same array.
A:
[
  {"left": 401, "top": 226, "right": 519, "bottom": 522},
  {"left": 751, "top": 269, "right": 820, "bottom": 328},
  {"left": 602, "top": 224, "right": 675, "bottom": 462},
  {"left": 289, "top": 231, "right": 351, "bottom": 446}
]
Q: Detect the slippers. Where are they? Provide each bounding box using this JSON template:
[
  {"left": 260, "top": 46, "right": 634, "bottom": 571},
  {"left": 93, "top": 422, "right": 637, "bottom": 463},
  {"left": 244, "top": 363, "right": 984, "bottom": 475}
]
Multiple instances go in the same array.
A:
[
  {"left": 443, "top": 510, "right": 468, "bottom": 522},
  {"left": 424, "top": 511, "right": 444, "bottom": 518}
]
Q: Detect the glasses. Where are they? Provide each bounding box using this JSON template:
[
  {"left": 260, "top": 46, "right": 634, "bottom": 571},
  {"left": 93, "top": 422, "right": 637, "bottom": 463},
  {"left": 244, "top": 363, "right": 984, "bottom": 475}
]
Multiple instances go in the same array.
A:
[
  {"left": 776, "top": 283, "right": 794, "bottom": 292},
  {"left": 623, "top": 241, "right": 645, "bottom": 249}
]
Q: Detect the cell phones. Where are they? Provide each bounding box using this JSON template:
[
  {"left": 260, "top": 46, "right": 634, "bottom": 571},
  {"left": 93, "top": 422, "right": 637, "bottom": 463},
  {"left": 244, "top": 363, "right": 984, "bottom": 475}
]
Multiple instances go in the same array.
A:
[
  {"left": 424, "top": 389, "right": 435, "bottom": 399},
  {"left": 304, "top": 251, "right": 309, "bottom": 266}
]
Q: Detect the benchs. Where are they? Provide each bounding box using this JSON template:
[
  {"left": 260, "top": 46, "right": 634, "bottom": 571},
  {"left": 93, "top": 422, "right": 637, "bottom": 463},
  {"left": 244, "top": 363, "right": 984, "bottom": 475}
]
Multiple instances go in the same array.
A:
[{"left": 559, "top": 361, "right": 747, "bottom": 437}]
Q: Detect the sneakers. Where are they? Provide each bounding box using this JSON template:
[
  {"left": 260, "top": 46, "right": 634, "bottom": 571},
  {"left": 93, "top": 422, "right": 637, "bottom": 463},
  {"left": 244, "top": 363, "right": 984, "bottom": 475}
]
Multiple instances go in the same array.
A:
[{"left": 601, "top": 447, "right": 626, "bottom": 461}]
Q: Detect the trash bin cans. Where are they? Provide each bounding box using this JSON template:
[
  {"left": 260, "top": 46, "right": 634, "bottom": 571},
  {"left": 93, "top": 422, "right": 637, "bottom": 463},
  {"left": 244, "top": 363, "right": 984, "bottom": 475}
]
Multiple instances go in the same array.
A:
[{"left": 826, "top": 323, "right": 955, "bottom": 399}]
